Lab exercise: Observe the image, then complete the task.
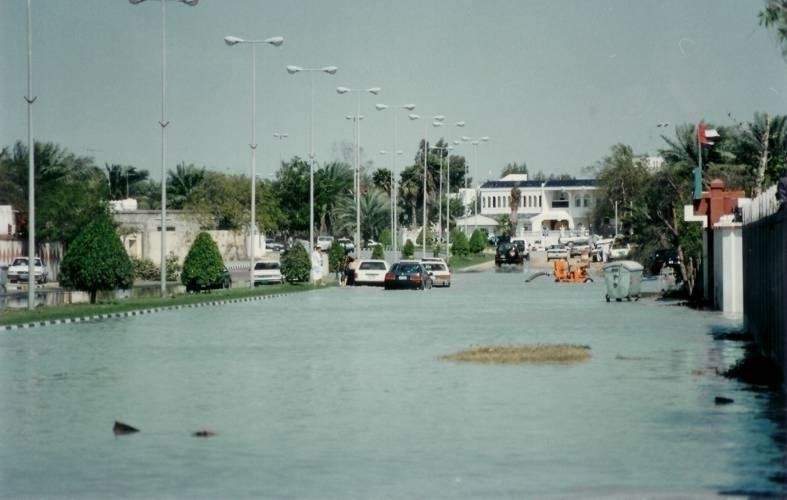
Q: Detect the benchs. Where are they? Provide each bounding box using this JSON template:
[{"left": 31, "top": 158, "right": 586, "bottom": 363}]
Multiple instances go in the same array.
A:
[{"left": 699, "top": 121, "right": 719, "bottom": 146}]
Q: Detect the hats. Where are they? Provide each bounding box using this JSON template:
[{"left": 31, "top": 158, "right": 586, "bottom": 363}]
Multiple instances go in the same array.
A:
[
  {"left": 6, "top": 255, "right": 46, "bottom": 286},
  {"left": 255, "top": 233, "right": 454, "bottom": 286},
  {"left": 649, "top": 250, "right": 681, "bottom": 275}
]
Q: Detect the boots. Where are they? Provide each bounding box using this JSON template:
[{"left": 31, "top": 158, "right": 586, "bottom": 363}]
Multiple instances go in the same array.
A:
[{"left": 491, "top": 232, "right": 633, "bottom": 270}]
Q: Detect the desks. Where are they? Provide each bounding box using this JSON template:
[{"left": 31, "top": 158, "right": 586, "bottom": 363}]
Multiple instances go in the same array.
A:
[
  {"left": 220, "top": 32, "right": 289, "bottom": 293},
  {"left": 273, "top": 132, "right": 290, "bottom": 171},
  {"left": 654, "top": 119, "right": 671, "bottom": 156},
  {"left": 284, "top": 64, "right": 338, "bottom": 253},
  {"left": 324, "top": 85, "right": 490, "bottom": 264},
  {"left": 121, "top": 0, "right": 205, "bottom": 299}
]
